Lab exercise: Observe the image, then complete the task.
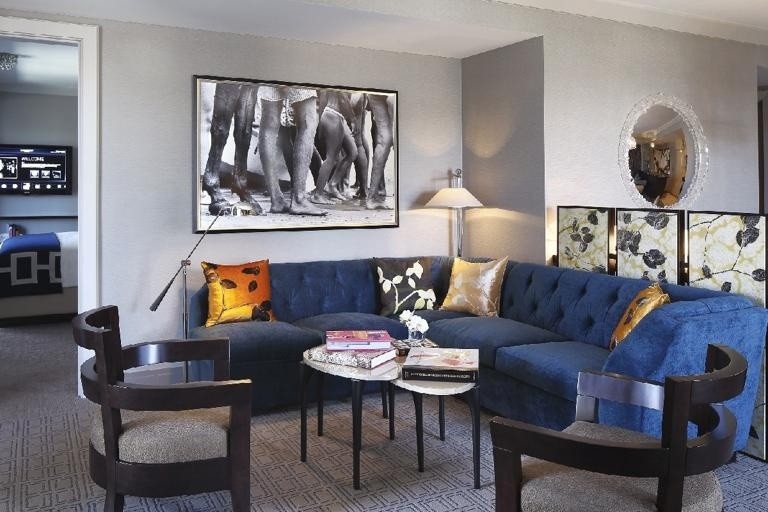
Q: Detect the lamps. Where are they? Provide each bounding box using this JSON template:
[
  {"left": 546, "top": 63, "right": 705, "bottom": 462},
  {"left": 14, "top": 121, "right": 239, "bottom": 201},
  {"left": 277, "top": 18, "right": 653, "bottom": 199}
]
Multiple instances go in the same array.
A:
[{"left": 426, "top": 170, "right": 483, "bottom": 253}]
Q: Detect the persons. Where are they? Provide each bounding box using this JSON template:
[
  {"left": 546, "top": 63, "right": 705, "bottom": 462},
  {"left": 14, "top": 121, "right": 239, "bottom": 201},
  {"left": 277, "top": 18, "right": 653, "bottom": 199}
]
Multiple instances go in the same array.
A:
[
  {"left": 366, "top": 92, "right": 395, "bottom": 211},
  {"left": 279, "top": 98, "right": 322, "bottom": 196},
  {"left": 349, "top": 90, "right": 369, "bottom": 204},
  {"left": 310, "top": 85, "right": 358, "bottom": 205},
  {"left": 257, "top": 83, "right": 329, "bottom": 216}
]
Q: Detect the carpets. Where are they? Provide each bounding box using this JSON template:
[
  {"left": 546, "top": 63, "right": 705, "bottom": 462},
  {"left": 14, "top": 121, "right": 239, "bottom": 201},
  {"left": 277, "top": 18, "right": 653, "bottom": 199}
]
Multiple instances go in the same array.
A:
[{"left": 1, "top": 314, "right": 768, "bottom": 509}]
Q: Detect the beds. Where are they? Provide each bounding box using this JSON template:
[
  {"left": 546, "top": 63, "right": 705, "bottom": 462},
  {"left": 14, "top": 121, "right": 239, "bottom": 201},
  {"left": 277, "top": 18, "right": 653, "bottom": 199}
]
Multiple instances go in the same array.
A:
[{"left": 0, "top": 230, "right": 78, "bottom": 324}]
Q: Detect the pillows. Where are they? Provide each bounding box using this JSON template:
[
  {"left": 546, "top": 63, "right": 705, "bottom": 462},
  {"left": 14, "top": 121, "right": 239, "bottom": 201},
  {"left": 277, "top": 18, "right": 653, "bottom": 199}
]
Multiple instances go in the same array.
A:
[
  {"left": 609, "top": 283, "right": 671, "bottom": 353},
  {"left": 373, "top": 257, "right": 438, "bottom": 313},
  {"left": 439, "top": 256, "right": 507, "bottom": 317},
  {"left": 197, "top": 262, "right": 276, "bottom": 323}
]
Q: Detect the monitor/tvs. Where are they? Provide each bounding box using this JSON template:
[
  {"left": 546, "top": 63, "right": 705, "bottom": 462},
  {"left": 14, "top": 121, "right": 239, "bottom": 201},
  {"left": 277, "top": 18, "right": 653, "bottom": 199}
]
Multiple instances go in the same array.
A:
[{"left": 0, "top": 144, "right": 73, "bottom": 195}]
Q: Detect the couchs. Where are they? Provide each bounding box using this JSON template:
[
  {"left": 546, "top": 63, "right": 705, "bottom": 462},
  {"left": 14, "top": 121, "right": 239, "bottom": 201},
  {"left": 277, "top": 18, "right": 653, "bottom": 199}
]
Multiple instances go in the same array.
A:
[{"left": 188, "top": 256, "right": 764, "bottom": 466}]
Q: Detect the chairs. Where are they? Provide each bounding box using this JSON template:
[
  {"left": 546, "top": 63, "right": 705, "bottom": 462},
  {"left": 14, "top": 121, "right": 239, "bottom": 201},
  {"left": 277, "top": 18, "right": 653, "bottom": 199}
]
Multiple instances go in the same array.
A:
[
  {"left": 490, "top": 344, "right": 748, "bottom": 512},
  {"left": 71, "top": 304, "right": 250, "bottom": 510}
]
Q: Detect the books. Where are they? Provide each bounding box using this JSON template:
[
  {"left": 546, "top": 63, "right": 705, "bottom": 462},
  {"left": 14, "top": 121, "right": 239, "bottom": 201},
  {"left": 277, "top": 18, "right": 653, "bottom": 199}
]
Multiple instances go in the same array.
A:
[
  {"left": 307, "top": 344, "right": 398, "bottom": 370},
  {"left": 325, "top": 330, "right": 392, "bottom": 353},
  {"left": 402, "top": 347, "right": 480, "bottom": 383},
  {"left": 390, "top": 339, "right": 439, "bottom": 357}
]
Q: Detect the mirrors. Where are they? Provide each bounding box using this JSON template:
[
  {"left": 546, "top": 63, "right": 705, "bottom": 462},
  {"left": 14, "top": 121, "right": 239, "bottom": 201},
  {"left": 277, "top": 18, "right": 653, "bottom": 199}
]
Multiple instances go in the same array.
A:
[{"left": 617, "top": 91, "right": 709, "bottom": 217}]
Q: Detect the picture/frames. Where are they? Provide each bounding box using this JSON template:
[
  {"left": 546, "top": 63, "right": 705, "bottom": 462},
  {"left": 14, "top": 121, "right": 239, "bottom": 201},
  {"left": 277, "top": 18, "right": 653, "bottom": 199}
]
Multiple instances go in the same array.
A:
[{"left": 191, "top": 74, "right": 402, "bottom": 229}]
